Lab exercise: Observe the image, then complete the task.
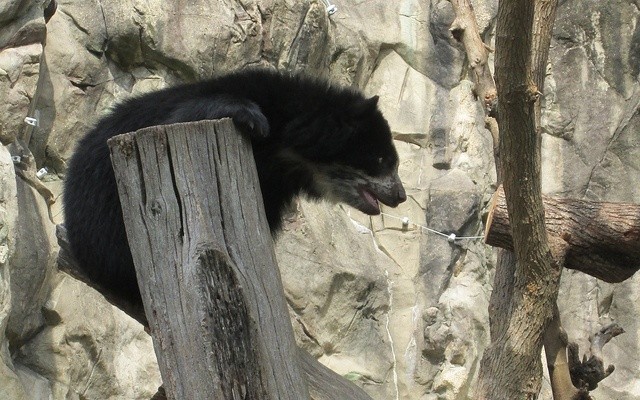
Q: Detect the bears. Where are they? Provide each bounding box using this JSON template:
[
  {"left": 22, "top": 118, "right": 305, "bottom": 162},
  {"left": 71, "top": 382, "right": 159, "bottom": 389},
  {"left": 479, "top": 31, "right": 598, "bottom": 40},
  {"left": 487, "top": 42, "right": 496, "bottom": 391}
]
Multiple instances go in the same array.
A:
[{"left": 60, "top": 67, "right": 407, "bottom": 309}]
